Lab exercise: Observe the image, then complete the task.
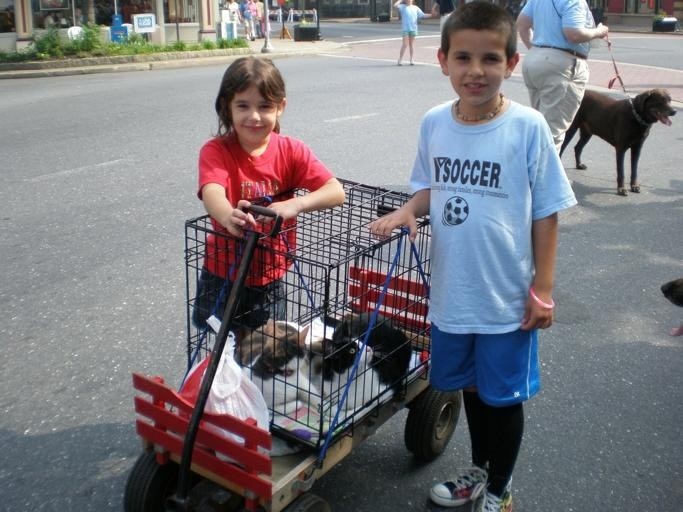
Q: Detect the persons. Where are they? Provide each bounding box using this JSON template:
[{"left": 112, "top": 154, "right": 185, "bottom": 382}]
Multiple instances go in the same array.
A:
[
  {"left": 74, "top": 7, "right": 84, "bottom": 26},
  {"left": 224, "top": 0, "right": 266, "bottom": 43},
  {"left": 286, "top": 8, "right": 294, "bottom": 22},
  {"left": 514, "top": 0, "right": 609, "bottom": 159},
  {"left": 393, "top": 1, "right": 437, "bottom": 68},
  {"left": 368, "top": 0, "right": 579, "bottom": 511},
  {"left": 189, "top": 56, "right": 349, "bottom": 339},
  {"left": 431, "top": 1, "right": 456, "bottom": 37},
  {"left": 42, "top": 11, "right": 58, "bottom": 30}
]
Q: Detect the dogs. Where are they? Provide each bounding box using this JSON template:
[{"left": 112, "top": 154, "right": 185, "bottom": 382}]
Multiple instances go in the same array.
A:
[{"left": 558, "top": 88, "right": 677, "bottom": 197}]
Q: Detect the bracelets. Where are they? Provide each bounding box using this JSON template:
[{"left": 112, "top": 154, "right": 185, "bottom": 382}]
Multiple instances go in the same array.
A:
[{"left": 528, "top": 286, "right": 556, "bottom": 313}]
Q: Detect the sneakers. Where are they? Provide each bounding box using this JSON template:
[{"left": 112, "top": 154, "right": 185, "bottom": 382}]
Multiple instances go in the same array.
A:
[
  {"left": 429, "top": 464, "right": 488, "bottom": 507},
  {"left": 475, "top": 487, "right": 513, "bottom": 511}
]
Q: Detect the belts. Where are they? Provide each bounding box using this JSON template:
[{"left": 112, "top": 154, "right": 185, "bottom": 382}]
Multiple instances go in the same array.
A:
[{"left": 535, "top": 44, "right": 587, "bottom": 60}]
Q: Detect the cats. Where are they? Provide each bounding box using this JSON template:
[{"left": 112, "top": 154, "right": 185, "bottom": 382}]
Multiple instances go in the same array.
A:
[
  {"left": 299, "top": 315, "right": 381, "bottom": 410},
  {"left": 233, "top": 318, "right": 342, "bottom": 418},
  {"left": 321, "top": 312, "right": 413, "bottom": 414}
]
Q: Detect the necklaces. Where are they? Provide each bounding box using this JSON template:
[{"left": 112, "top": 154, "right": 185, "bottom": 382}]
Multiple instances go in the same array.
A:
[{"left": 452, "top": 93, "right": 504, "bottom": 122}]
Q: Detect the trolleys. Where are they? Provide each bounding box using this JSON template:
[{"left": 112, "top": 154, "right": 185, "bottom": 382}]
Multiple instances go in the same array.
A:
[{"left": 111, "top": 167, "right": 467, "bottom": 511}]
